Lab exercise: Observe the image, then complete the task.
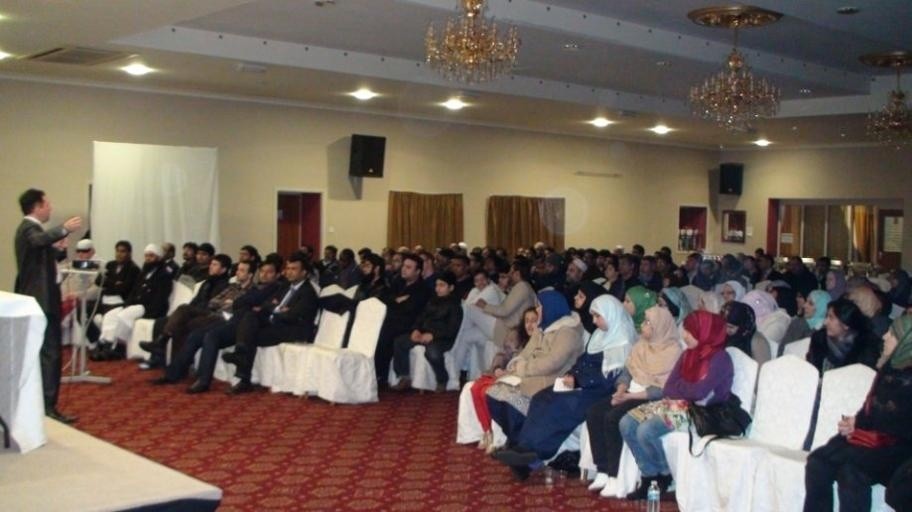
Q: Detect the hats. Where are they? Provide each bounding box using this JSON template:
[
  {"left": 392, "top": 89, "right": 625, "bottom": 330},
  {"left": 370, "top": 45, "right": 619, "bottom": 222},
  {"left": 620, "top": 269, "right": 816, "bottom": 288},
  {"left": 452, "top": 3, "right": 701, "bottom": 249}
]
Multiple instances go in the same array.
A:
[
  {"left": 144, "top": 243, "right": 161, "bottom": 258},
  {"left": 573, "top": 258, "right": 589, "bottom": 275}
]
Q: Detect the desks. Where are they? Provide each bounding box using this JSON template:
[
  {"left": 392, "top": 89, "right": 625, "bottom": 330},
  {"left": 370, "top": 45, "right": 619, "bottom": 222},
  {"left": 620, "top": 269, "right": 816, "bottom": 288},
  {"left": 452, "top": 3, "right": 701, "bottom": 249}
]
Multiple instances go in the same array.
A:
[
  {"left": 0, "top": 289, "right": 49, "bottom": 449},
  {"left": 58, "top": 266, "right": 114, "bottom": 385}
]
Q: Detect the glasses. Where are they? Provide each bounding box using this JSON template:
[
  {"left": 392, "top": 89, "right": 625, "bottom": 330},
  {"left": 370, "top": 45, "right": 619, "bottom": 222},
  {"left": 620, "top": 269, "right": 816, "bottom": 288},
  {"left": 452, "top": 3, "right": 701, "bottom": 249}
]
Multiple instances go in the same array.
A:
[{"left": 76, "top": 248, "right": 90, "bottom": 253}]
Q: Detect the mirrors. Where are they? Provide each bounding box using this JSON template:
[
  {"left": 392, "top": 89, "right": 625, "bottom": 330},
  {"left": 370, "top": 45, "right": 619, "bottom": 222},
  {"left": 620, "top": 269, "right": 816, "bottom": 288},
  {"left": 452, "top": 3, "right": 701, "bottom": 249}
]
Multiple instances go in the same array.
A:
[{"left": 720, "top": 210, "right": 746, "bottom": 244}]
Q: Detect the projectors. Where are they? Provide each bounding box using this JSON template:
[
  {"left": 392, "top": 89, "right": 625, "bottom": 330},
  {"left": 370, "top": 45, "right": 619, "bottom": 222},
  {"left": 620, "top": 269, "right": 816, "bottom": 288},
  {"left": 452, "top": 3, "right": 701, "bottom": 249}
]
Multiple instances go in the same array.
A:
[{"left": 73, "top": 259, "right": 101, "bottom": 271}]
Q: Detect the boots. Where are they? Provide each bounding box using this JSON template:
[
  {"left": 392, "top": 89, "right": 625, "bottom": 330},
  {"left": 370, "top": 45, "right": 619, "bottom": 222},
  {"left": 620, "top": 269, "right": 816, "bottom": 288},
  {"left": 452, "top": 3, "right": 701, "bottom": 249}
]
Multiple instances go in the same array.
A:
[
  {"left": 658, "top": 473, "right": 673, "bottom": 498},
  {"left": 89, "top": 342, "right": 112, "bottom": 360},
  {"left": 103, "top": 342, "right": 127, "bottom": 360},
  {"left": 627, "top": 475, "right": 658, "bottom": 500}
]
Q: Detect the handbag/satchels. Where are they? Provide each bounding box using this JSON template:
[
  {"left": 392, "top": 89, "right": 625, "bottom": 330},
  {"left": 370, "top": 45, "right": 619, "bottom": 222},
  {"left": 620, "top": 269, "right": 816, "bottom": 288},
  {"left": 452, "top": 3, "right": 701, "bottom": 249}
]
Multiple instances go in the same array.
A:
[{"left": 685, "top": 392, "right": 752, "bottom": 437}]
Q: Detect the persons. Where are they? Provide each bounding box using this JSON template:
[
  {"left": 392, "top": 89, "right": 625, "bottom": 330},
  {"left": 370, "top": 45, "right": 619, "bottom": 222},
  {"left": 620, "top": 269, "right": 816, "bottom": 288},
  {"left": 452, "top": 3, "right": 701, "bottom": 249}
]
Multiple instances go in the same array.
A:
[
  {"left": 13, "top": 188, "right": 84, "bottom": 423},
  {"left": 297, "top": 237, "right": 911, "bottom": 510},
  {"left": 59, "top": 238, "right": 321, "bottom": 396}
]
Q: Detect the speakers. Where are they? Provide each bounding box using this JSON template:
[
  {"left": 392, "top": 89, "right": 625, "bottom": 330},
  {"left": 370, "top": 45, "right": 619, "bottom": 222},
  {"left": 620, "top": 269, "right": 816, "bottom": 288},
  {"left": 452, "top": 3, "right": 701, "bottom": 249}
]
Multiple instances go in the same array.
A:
[
  {"left": 349, "top": 134, "right": 386, "bottom": 178},
  {"left": 719, "top": 163, "right": 744, "bottom": 195}
]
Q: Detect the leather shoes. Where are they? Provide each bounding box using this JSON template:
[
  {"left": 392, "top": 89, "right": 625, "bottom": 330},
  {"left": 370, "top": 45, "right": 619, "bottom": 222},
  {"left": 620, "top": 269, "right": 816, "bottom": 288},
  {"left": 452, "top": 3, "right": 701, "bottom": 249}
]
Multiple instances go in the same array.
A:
[
  {"left": 186, "top": 379, "right": 209, "bottom": 393},
  {"left": 391, "top": 380, "right": 411, "bottom": 390},
  {"left": 46, "top": 409, "right": 79, "bottom": 423},
  {"left": 485, "top": 429, "right": 495, "bottom": 455},
  {"left": 138, "top": 360, "right": 167, "bottom": 371},
  {"left": 221, "top": 352, "right": 248, "bottom": 366},
  {"left": 490, "top": 446, "right": 538, "bottom": 467},
  {"left": 150, "top": 375, "right": 183, "bottom": 383},
  {"left": 478, "top": 432, "right": 488, "bottom": 450},
  {"left": 434, "top": 376, "right": 448, "bottom": 393},
  {"left": 510, "top": 465, "right": 532, "bottom": 482},
  {"left": 225, "top": 381, "right": 255, "bottom": 394},
  {"left": 139, "top": 341, "right": 166, "bottom": 355}
]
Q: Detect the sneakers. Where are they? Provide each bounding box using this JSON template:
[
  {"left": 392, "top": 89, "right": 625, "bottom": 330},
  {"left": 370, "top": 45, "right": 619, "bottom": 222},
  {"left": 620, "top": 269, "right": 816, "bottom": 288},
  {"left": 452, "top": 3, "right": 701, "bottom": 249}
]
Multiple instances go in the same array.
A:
[
  {"left": 587, "top": 472, "right": 608, "bottom": 492},
  {"left": 600, "top": 477, "right": 616, "bottom": 498}
]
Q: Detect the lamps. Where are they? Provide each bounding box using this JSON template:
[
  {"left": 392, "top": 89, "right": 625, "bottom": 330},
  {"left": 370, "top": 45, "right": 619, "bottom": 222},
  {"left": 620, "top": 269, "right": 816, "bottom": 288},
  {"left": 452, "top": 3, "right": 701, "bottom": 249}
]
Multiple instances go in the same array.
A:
[
  {"left": 859, "top": 50, "right": 912, "bottom": 148},
  {"left": 682, "top": 3, "right": 786, "bottom": 133},
  {"left": 423, "top": 0, "right": 524, "bottom": 89}
]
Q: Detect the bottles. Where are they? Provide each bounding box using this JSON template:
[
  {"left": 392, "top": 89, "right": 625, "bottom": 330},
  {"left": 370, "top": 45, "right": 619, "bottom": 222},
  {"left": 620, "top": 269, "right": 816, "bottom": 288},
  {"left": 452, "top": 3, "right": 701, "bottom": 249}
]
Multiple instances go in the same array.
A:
[{"left": 647, "top": 479, "right": 661, "bottom": 512}]
